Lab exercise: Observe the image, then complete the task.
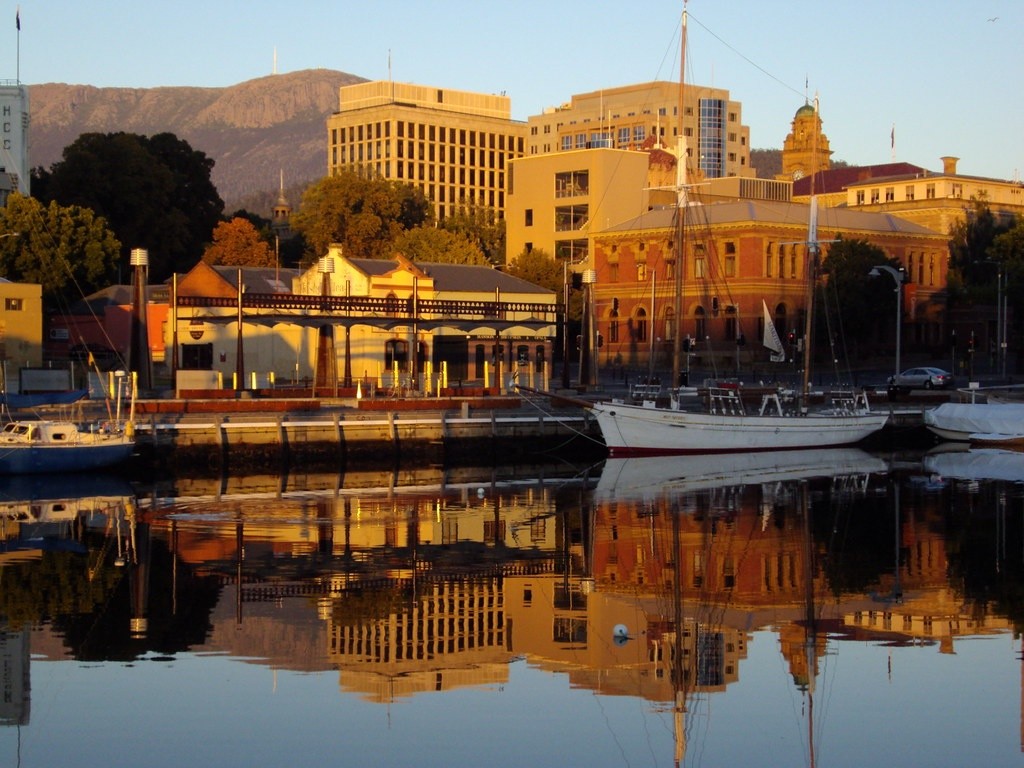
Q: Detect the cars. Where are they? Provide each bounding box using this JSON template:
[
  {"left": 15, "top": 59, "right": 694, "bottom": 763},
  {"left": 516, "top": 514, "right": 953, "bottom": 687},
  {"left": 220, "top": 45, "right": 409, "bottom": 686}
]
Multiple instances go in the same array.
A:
[{"left": 887, "top": 367, "right": 952, "bottom": 389}]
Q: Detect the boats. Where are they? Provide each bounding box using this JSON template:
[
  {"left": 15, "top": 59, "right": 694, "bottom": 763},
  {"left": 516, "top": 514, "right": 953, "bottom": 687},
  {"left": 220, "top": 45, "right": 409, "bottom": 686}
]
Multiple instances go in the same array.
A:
[
  {"left": 924, "top": 441, "right": 1024, "bottom": 484},
  {"left": 922, "top": 384, "right": 1024, "bottom": 449}
]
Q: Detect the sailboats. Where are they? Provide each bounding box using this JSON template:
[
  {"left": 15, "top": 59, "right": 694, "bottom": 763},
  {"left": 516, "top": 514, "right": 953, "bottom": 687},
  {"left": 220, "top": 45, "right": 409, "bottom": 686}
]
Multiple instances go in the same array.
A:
[
  {"left": 0, "top": 140, "right": 139, "bottom": 482},
  {"left": 0, "top": 474, "right": 137, "bottom": 768},
  {"left": 510, "top": 0, "right": 893, "bottom": 456},
  {"left": 518, "top": 439, "right": 891, "bottom": 768}
]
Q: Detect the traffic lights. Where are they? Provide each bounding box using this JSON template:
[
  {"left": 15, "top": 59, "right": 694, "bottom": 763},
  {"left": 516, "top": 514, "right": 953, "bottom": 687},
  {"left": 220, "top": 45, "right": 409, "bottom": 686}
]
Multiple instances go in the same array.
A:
[{"left": 789, "top": 333, "right": 794, "bottom": 345}]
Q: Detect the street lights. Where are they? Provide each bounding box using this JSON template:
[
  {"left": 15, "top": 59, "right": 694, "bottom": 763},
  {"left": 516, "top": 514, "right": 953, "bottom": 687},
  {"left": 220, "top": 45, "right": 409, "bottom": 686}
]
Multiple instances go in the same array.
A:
[{"left": 868, "top": 264, "right": 904, "bottom": 390}]
